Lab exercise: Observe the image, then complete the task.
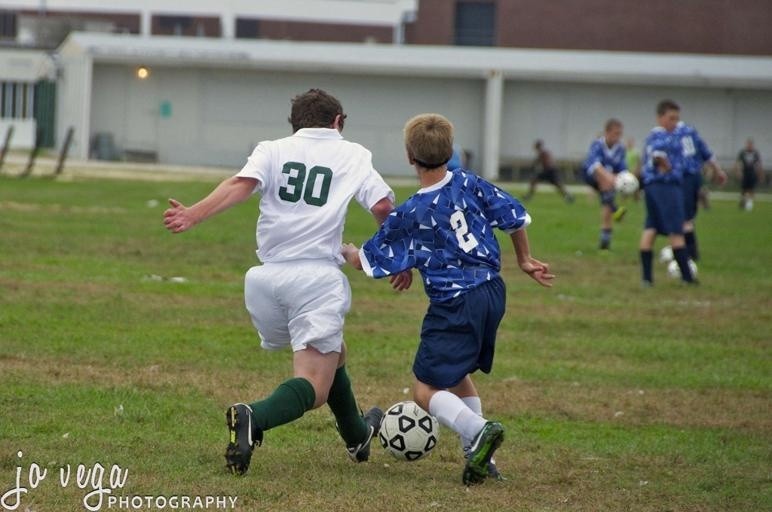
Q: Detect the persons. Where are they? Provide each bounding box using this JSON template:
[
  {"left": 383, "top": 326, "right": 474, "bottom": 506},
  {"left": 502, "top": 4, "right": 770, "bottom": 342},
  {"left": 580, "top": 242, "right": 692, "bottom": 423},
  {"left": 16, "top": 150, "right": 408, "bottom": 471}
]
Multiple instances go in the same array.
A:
[
  {"left": 582, "top": 97, "right": 728, "bottom": 291},
  {"left": 521, "top": 138, "right": 576, "bottom": 205},
  {"left": 339, "top": 112, "right": 556, "bottom": 488},
  {"left": 164, "top": 86, "right": 414, "bottom": 480},
  {"left": 731, "top": 135, "right": 765, "bottom": 212}
]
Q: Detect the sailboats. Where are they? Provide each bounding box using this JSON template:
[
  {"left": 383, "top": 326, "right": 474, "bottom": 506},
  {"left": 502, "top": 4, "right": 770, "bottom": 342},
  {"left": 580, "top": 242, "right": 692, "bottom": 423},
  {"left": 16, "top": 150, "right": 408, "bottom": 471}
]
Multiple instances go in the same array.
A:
[
  {"left": 661, "top": 244, "right": 676, "bottom": 262},
  {"left": 378, "top": 400, "right": 440, "bottom": 463},
  {"left": 614, "top": 169, "right": 640, "bottom": 197},
  {"left": 670, "top": 256, "right": 699, "bottom": 281}
]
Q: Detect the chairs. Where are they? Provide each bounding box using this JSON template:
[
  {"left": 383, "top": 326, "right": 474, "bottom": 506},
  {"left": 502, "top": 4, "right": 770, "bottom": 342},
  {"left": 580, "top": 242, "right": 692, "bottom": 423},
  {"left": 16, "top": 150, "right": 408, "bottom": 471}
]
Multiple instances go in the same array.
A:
[
  {"left": 522, "top": 195, "right": 701, "bottom": 289},
  {"left": 346, "top": 407, "right": 383, "bottom": 463},
  {"left": 462, "top": 420, "right": 505, "bottom": 486},
  {"left": 224, "top": 401, "right": 260, "bottom": 477}
]
